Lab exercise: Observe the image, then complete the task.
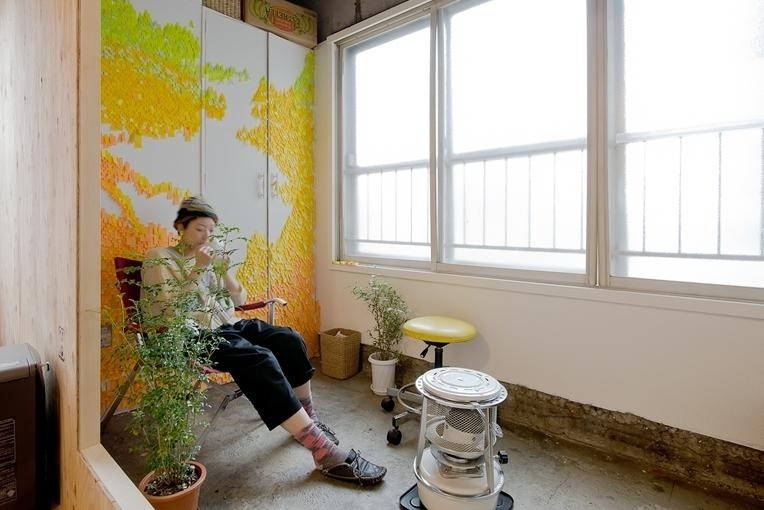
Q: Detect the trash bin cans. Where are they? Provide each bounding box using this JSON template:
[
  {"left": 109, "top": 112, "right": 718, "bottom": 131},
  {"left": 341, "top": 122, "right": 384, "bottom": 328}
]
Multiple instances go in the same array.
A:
[
  {"left": 0, "top": 341, "right": 50, "bottom": 510},
  {"left": 320, "top": 328, "right": 361, "bottom": 380}
]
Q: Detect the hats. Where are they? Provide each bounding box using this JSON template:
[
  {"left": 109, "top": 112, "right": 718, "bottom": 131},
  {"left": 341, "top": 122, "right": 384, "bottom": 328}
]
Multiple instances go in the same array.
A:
[{"left": 173, "top": 196, "right": 218, "bottom": 230}]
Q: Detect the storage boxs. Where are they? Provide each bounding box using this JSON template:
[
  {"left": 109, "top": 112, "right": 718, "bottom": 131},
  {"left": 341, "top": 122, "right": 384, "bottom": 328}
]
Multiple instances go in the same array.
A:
[{"left": 242, "top": 0, "right": 318, "bottom": 49}]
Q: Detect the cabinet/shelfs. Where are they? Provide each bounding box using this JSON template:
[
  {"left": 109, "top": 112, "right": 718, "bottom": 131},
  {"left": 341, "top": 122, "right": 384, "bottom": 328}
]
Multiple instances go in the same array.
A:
[{"left": 203, "top": 6, "right": 320, "bottom": 390}]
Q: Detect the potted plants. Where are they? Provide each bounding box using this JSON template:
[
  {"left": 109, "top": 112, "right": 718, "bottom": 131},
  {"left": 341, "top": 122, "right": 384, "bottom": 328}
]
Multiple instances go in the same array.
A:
[
  {"left": 79, "top": 220, "right": 255, "bottom": 510},
  {"left": 343, "top": 273, "right": 414, "bottom": 393}
]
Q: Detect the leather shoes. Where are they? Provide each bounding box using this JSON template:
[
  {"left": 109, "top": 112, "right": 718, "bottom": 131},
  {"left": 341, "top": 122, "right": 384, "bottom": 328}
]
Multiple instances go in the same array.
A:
[
  {"left": 320, "top": 448, "right": 387, "bottom": 482},
  {"left": 289, "top": 421, "right": 339, "bottom": 447}
]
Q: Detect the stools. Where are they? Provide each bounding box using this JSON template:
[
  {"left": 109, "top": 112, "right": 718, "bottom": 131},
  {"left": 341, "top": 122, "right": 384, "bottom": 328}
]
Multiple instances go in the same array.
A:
[{"left": 380, "top": 316, "right": 477, "bottom": 445}]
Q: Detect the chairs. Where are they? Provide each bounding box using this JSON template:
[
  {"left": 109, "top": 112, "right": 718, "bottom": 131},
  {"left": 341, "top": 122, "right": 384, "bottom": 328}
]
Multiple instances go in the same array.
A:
[{"left": 101, "top": 255, "right": 288, "bottom": 461}]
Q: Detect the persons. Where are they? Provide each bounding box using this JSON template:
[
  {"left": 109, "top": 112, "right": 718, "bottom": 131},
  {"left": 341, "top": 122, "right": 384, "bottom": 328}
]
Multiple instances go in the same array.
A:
[{"left": 139, "top": 194, "right": 386, "bottom": 483}]
